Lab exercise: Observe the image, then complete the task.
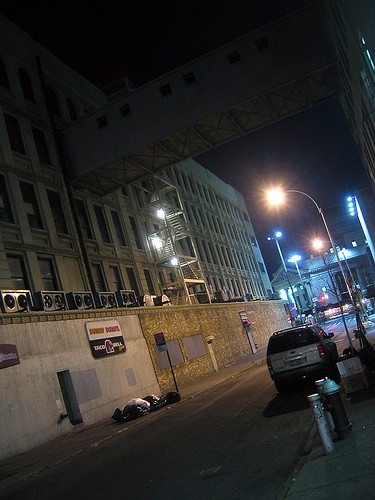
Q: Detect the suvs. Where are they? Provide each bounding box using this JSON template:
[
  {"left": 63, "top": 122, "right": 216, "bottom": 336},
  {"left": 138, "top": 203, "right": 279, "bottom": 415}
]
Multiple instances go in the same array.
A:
[{"left": 266, "top": 323, "right": 339, "bottom": 394}]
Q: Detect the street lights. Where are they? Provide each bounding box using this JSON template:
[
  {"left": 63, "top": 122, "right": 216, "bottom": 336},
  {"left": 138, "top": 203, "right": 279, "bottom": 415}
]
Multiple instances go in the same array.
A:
[
  {"left": 285, "top": 254, "right": 316, "bottom": 310},
  {"left": 265, "top": 228, "right": 307, "bottom": 326},
  {"left": 259, "top": 183, "right": 368, "bottom": 350},
  {"left": 309, "top": 231, "right": 360, "bottom": 289}
]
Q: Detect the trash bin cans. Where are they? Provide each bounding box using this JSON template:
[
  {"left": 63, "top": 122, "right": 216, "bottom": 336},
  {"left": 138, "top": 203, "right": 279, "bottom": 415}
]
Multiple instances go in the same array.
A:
[{"left": 336, "top": 354, "right": 370, "bottom": 398}]
